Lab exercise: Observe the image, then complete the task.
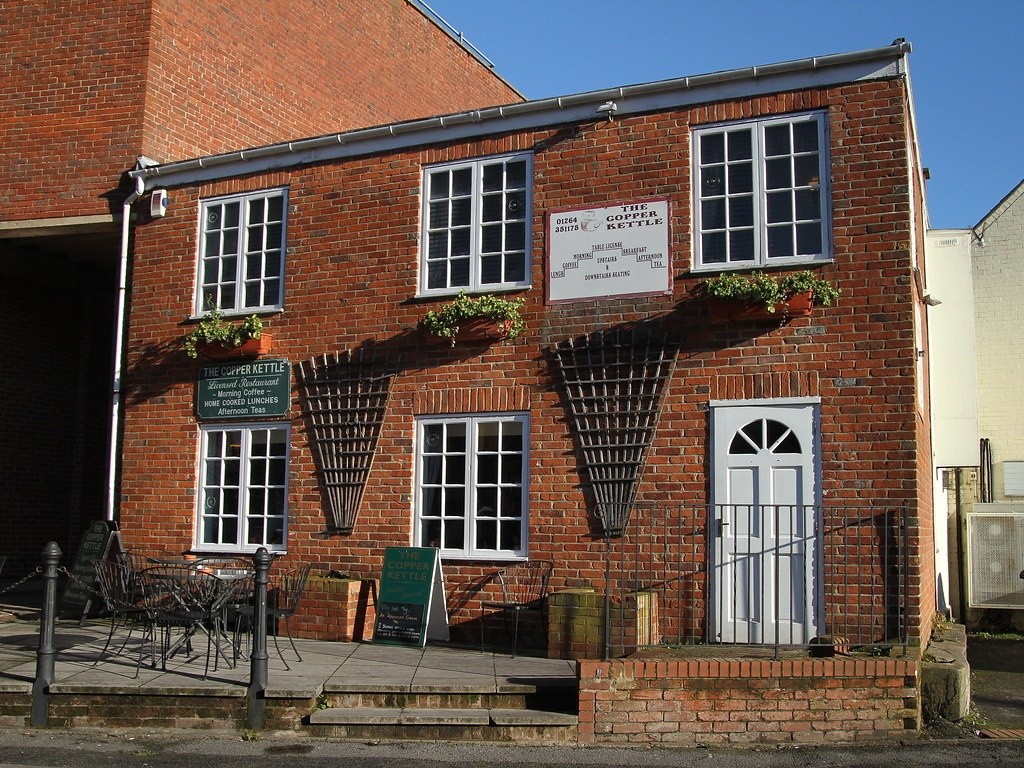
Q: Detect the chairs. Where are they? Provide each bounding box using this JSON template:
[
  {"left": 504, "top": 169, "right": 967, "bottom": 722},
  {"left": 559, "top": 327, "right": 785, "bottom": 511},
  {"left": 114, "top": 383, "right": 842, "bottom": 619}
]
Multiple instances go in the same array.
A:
[
  {"left": 91, "top": 546, "right": 313, "bottom": 681},
  {"left": 480, "top": 560, "right": 554, "bottom": 657}
]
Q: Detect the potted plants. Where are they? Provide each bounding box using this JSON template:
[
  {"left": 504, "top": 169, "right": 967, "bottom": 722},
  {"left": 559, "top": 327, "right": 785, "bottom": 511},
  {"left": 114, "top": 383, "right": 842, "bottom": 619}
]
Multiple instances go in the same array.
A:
[
  {"left": 421, "top": 290, "right": 529, "bottom": 352},
  {"left": 186, "top": 294, "right": 274, "bottom": 360},
  {"left": 705, "top": 269, "right": 842, "bottom": 326}
]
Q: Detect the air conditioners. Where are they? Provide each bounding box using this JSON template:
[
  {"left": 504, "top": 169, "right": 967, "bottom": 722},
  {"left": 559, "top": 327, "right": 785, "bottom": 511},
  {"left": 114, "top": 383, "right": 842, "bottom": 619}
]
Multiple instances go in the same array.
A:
[{"left": 966, "top": 512, "right": 1024, "bottom": 609}]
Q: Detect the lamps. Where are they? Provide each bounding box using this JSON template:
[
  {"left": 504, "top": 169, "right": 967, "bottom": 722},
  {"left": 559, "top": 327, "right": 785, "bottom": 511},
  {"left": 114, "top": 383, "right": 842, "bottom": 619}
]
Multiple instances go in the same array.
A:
[{"left": 596, "top": 101, "right": 618, "bottom": 122}]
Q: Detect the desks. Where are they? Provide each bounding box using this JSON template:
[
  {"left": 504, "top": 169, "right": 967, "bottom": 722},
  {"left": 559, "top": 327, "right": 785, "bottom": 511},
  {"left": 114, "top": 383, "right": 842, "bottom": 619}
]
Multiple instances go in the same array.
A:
[
  {"left": 147, "top": 569, "right": 249, "bottom": 669},
  {"left": 148, "top": 555, "right": 244, "bottom": 576}
]
